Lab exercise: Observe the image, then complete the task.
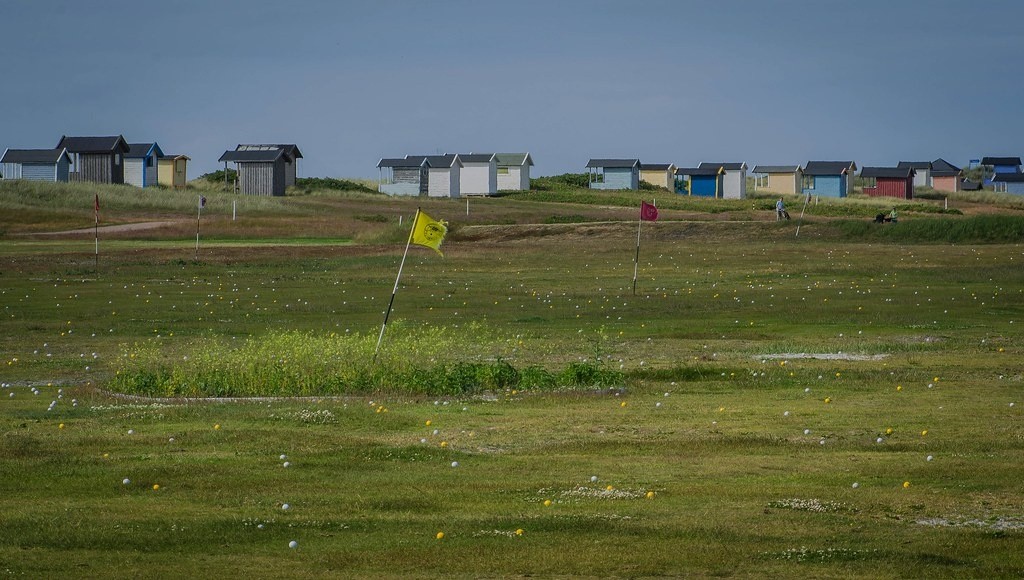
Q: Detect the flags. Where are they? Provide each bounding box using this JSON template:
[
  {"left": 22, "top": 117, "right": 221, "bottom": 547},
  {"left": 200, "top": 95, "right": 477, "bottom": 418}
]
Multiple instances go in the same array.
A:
[
  {"left": 640, "top": 200, "right": 659, "bottom": 221},
  {"left": 408, "top": 209, "right": 450, "bottom": 258},
  {"left": 95, "top": 194, "right": 100, "bottom": 211},
  {"left": 804, "top": 191, "right": 811, "bottom": 204},
  {"left": 199, "top": 194, "right": 207, "bottom": 208}
]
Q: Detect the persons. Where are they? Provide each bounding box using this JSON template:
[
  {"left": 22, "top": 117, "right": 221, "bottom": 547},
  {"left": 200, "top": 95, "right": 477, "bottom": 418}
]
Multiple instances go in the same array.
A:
[
  {"left": 889, "top": 206, "right": 898, "bottom": 220},
  {"left": 776, "top": 197, "right": 784, "bottom": 221}
]
